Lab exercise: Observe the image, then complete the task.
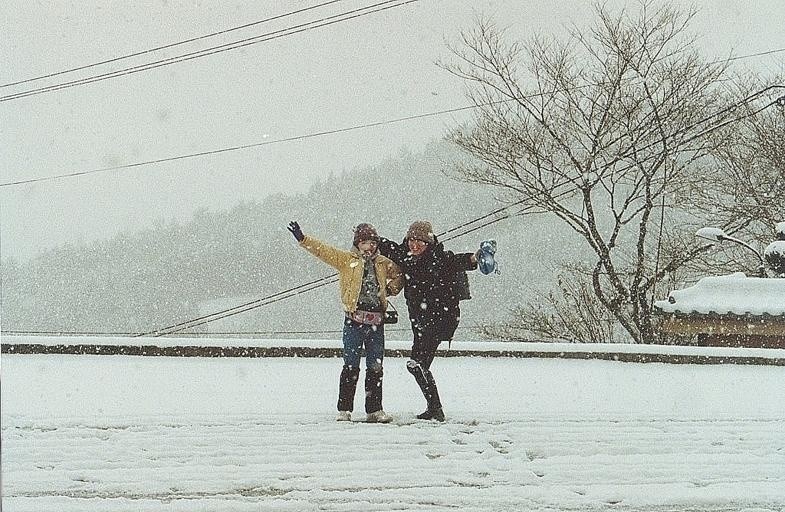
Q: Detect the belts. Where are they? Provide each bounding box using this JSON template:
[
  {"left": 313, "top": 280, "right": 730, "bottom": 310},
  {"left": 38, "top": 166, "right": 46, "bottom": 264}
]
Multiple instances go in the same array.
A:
[{"left": 345, "top": 310, "right": 382, "bottom": 325}]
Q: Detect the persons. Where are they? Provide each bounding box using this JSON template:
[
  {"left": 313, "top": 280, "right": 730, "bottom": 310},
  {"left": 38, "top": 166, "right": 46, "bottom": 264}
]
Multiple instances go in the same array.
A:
[
  {"left": 287, "top": 219, "right": 405, "bottom": 422},
  {"left": 379, "top": 220, "right": 497, "bottom": 422}
]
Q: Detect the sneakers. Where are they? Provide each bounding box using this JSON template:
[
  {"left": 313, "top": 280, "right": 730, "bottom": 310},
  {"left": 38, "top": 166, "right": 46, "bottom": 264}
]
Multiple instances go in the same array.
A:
[
  {"left": 367, "top": 410, "right": 393, "bottom": 423},
  {"left": 336, "top": 410, "right": 351, "bottom": 421},
  {"left": 417, "top": 408, "right": 445, "bottom": 422}
]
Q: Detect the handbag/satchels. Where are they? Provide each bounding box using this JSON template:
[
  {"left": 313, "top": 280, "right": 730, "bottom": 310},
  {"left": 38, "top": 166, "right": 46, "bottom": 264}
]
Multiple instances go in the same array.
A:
[{"left": 383, "top": 300, "right": 398, "bottom": 324}]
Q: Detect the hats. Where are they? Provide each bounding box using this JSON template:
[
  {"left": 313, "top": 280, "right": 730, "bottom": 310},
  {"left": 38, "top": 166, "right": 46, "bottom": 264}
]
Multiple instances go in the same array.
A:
[
  {"left": 353, "top": 223, "right": 381, "bottom": 246},
  {"left": 407, "top": 221, "right": 435, "bottom": 245}
]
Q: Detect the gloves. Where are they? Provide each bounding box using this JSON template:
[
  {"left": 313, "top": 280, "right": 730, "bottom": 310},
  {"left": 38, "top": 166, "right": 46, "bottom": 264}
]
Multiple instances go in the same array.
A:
[{"left": 287, "top": 221, "right": 305, "bottom": 242}]
